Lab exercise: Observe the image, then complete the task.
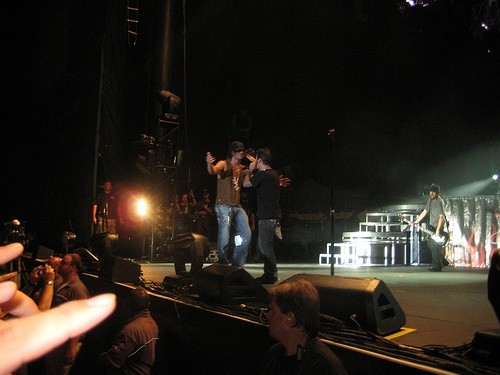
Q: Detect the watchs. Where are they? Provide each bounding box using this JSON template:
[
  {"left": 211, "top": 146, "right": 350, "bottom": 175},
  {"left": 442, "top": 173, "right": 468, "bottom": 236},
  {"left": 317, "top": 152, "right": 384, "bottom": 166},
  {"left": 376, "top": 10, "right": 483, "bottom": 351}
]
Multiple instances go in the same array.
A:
[{"left": 45, "top": 279, "right": 55, "bottom": 286}]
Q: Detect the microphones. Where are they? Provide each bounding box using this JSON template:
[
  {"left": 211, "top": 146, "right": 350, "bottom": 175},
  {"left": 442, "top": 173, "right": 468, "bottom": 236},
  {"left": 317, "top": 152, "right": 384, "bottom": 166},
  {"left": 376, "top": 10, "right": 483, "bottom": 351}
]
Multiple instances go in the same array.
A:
[
  {"left": 242, "top": 166, "right": 249, "bottom": 170},
  {"left": 328, "top": 129, "right": 335, "bottom": 136}
]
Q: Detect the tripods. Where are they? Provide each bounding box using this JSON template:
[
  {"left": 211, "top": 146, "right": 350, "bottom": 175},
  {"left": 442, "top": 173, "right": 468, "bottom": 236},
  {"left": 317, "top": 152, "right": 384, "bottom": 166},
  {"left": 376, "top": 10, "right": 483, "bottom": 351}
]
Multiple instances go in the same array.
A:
[{"left": 9, "top": 258, "right": 30, "bottom": 289}]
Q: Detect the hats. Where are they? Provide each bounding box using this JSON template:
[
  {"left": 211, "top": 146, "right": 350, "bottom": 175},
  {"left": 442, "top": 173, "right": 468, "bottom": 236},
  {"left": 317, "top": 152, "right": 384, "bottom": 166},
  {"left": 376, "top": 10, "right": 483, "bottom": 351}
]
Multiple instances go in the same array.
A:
[
  {"left": 429, "top": 184, "right": 440, "bottom": 194},
  {"left": 230, "top": 141, "right": 245, "bottom": 152}
]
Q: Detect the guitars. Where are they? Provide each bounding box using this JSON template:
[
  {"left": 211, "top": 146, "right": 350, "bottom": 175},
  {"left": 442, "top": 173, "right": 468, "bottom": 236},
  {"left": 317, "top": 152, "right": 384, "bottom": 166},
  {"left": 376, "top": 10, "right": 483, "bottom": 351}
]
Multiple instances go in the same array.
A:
[{"left": 397, "top": 214, "right": 449, "bottom": 246}]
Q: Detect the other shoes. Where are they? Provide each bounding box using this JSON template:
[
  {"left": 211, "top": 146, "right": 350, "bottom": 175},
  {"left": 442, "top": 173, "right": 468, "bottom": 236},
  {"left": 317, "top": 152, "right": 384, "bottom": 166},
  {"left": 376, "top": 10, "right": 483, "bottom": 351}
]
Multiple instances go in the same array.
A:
[
  {"left": 441, "top": 262, "right": 449, "bottom": 267},
  {"left": 255, "top": 276, "right": 277, "bottom": 284},
  {"left": 429, "top": 268, "right": 441, "bottom": 272}
]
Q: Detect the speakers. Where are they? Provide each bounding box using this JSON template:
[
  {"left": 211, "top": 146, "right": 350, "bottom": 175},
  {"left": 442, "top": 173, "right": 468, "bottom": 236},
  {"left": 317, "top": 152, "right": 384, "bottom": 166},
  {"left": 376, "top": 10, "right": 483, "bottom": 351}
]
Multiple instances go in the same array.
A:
[
  {"left": 197, "top": 263, "right": 269, "bottom": 306},
  {"left": 73, "top": 248, "right": 99, "bottom": 263},
  {"left": 279, "top": 273, "right": 406, "bottom": 335},
  {"left": 99, "top": 257, "right": 141, "bottom": 283},
  {"left": 32, "top": 245, "right": 54, "bottom": 259}
]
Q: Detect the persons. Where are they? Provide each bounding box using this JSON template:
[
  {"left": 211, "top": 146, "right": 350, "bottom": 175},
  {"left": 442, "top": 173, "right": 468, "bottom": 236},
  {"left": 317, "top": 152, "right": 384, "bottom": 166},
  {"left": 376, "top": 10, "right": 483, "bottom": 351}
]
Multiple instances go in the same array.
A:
[
  {"left": 407, "top": 184, "right": 449, "bottom": 272},
  {"left": 206, "top": 141, "right": 291, "bottom": 284},
  {"left": 92, "top": 180, "right": 257, "bottom": 262},
  {"left": 1, "top": 236, "right": 345, "bottom": 375}
]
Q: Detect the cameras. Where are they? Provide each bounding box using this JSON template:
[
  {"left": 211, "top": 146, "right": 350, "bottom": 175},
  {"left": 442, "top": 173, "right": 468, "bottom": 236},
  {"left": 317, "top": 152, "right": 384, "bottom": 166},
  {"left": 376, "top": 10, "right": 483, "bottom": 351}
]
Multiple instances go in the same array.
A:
[{"left": 38, "top": 268, "right": 54, "bottom": 277}]
